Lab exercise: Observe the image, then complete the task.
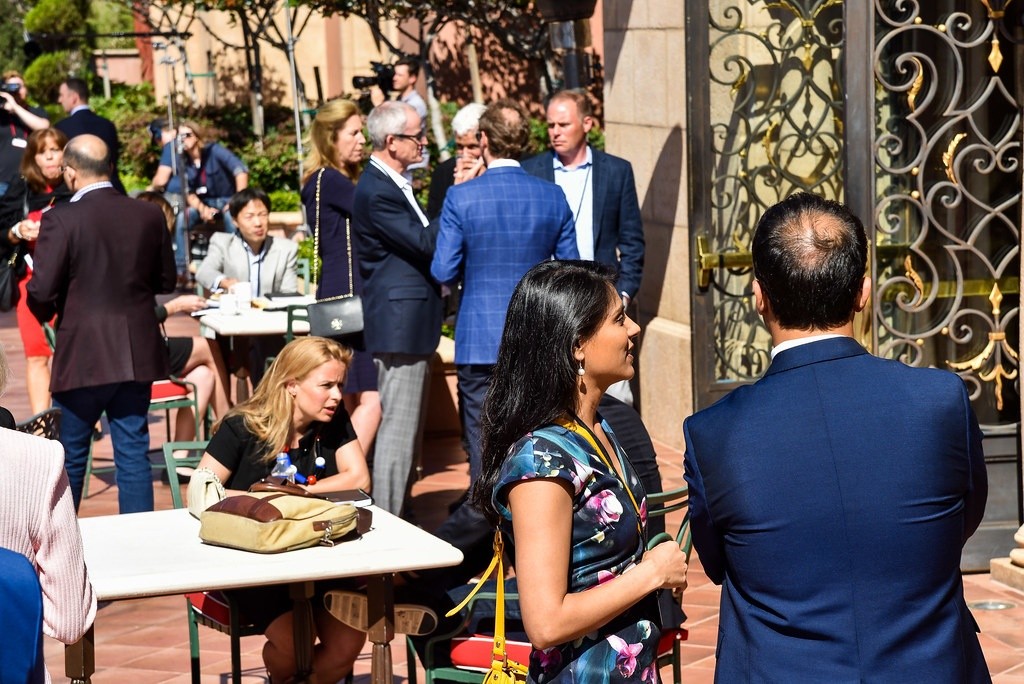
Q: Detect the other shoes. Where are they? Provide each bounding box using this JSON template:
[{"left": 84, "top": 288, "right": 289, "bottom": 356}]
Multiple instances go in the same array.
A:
[
  {"left": 177, "top": 273, "right": 190, "bottom": 289},
  {"left": 163, "top": 462, "right": 194, "bottom": 485},
  {"left": 322, "top": 593, "right": 438, "bottom": 636}
]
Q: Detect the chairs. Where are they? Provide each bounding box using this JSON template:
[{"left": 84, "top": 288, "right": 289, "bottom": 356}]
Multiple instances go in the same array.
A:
[
  {"left": 163, "top": 440, "right": 295, "bottom": 684},
  {"left": 424, "top": 488, "right": 698, "bottom": 684},
  {"left": 42, "top": 322, "right": 199, "bottom": 498}
]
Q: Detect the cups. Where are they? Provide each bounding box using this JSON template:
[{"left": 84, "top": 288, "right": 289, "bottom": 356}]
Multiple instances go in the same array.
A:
[
  {"left": 220, "top": 294, "right": 236, "bottom": 315},
  {"left": 235, "top": 282, "right": 251, "bottom": 311}
]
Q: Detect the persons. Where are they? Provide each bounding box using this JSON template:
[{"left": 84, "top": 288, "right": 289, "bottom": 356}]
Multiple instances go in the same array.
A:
[
  {"left": 519, "top": 89, "right": 646, "bottom": 410},
  {"left": 0, "top": 71, "right": 249, "bottom": 484},
  {"left": 193, "top": 188, "right": 303, "bottom": 390},
  {"left": 0, "top": 340, "right": 97, "bottom": 683},
  {"left": 323, "top": 392, "right": 687, "bottom": 684},
  {"left": 471, "top": 258, "right": 688, "bottom": 683},
  {"left": 183, "top": 336, "right": 370, "bottom": 683},
  {"left": 367, "top": 56, "right": 429, "bottom": 187},
  {"left": 425, "top": 101, "right": 489, "bottom": 220},
  {"left": 24, "top": 134, "right": 177, "bottom": 519},
  {"left": 300, "top": 97, "right": 384, "bottom": 458},
  {"left": 681, "top": 194, "right": 991, "bottom": 684},
  {"left": 429, "top": 98, "right": 582, "bottom": 487},
  {"left": 351, "top": 99, "right": 450, "bottom": 517}
]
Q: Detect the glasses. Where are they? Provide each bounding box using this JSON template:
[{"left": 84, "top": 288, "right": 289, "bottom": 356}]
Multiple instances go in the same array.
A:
[
  {"left": 476, "top": 133, "right": 483, "bottom": 141},
  {"left": 186, "top": 133, "right": 195, "bottom": 138}
]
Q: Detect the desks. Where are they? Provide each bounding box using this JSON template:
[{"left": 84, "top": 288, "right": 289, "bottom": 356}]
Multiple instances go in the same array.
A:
[
  {"left": 200, "top": 307, "right": 311, "bottom": 405},
  {"left": 76, "top": 503, "right": 464, "bottom": 684}
]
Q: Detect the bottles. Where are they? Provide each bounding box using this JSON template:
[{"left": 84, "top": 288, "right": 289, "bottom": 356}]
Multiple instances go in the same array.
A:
[{"left": 271, "top": 452, "right": 296, "bottom": 484}]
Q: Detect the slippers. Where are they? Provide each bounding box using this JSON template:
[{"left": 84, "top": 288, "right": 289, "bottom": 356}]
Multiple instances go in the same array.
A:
[{"left": 396, "top": 129, "right": 423, "bottom": 141}]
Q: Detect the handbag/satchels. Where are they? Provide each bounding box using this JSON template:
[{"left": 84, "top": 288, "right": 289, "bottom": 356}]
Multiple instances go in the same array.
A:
[
  {"left": 198, "top": 484, "right": 374, "bottom": 555},
  {"left": 483, "top": 659, "right": 529, "bottom": 684},
  {"left": 307, "top": 295, "right": 365, "bottom": 337},
  {"left": 0, "top": 261, "right": 20, "bottom": 312}
]
